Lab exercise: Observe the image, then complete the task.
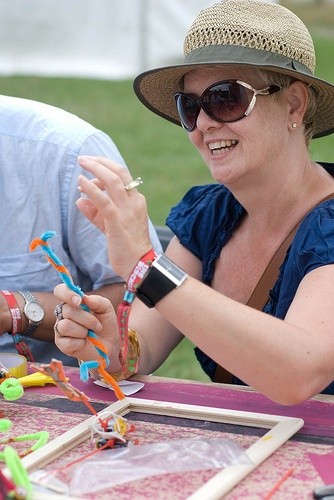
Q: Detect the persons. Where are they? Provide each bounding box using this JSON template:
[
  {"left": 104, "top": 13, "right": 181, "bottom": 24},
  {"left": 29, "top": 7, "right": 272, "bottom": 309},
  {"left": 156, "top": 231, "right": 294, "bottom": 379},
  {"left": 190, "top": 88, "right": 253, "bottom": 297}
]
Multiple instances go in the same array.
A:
[
  {"left": 54, "top": 0, "right": 334, "bottom": 405},
  {"left": 0, "top": 95, "right": 164, "bottom": 366}
]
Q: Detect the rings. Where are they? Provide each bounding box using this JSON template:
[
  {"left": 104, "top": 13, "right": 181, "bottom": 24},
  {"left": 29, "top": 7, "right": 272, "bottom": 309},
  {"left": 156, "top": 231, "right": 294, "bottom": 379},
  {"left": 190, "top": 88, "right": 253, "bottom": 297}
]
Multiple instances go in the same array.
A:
[
  {"left": 55, "top": 321, "right": 63, "bottom": 337},
  {"left": 125, "top": 176, "right": 143, "bottom": 190},
  {"left": 54, "top": 302, "right": 66, "bottom": 320}
]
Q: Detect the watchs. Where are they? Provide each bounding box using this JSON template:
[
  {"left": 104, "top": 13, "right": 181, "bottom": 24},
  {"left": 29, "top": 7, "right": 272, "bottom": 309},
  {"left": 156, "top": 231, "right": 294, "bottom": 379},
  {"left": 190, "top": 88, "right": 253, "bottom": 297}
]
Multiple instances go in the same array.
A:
[
  {"left": 136, "top": 252, "right": 189, "bottom": 309},
  {"left": 19, "top": 291, "right": 46, "bottom": 336}
]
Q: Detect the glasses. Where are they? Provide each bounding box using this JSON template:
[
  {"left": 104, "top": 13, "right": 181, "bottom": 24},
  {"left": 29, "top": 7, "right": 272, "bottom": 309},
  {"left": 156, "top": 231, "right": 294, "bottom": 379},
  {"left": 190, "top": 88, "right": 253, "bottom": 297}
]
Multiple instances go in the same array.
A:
[{"left": 173, "top": 78, "right": 298, "bottom": 131}]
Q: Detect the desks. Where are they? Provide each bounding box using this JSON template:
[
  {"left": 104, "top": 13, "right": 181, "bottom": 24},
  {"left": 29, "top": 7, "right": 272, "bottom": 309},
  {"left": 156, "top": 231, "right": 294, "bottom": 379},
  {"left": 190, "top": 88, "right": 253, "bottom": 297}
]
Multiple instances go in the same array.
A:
[{"left": 0, "top": 348, "right": 334, "bottom": 500}]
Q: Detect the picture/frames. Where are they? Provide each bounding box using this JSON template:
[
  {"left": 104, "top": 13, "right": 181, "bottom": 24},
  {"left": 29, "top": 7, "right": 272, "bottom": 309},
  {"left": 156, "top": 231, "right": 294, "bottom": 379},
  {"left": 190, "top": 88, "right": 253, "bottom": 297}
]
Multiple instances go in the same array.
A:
[{"left": 2, "top": 398, "right": 305, "bottom": 500}]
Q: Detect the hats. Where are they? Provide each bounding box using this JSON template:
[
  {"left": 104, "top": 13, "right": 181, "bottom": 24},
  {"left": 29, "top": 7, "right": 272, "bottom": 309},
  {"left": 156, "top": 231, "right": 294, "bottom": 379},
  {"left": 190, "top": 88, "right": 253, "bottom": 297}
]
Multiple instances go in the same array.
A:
[{"left": 133, "top": 0, "right": 334, "bottom": 139}]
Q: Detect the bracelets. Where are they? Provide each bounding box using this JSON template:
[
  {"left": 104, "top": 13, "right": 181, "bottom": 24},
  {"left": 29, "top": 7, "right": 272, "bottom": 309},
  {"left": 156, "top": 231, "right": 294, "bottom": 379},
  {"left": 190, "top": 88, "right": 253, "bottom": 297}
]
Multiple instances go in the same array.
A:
[
  {"left": 118, "top": 249, "right": 159, "bottom": 374},
  {"left": 0, "top": 290, "right": 34, "bottom": 361},
  {"left": 77, "top": 329, "right": 141, "bottom": 384}
]
